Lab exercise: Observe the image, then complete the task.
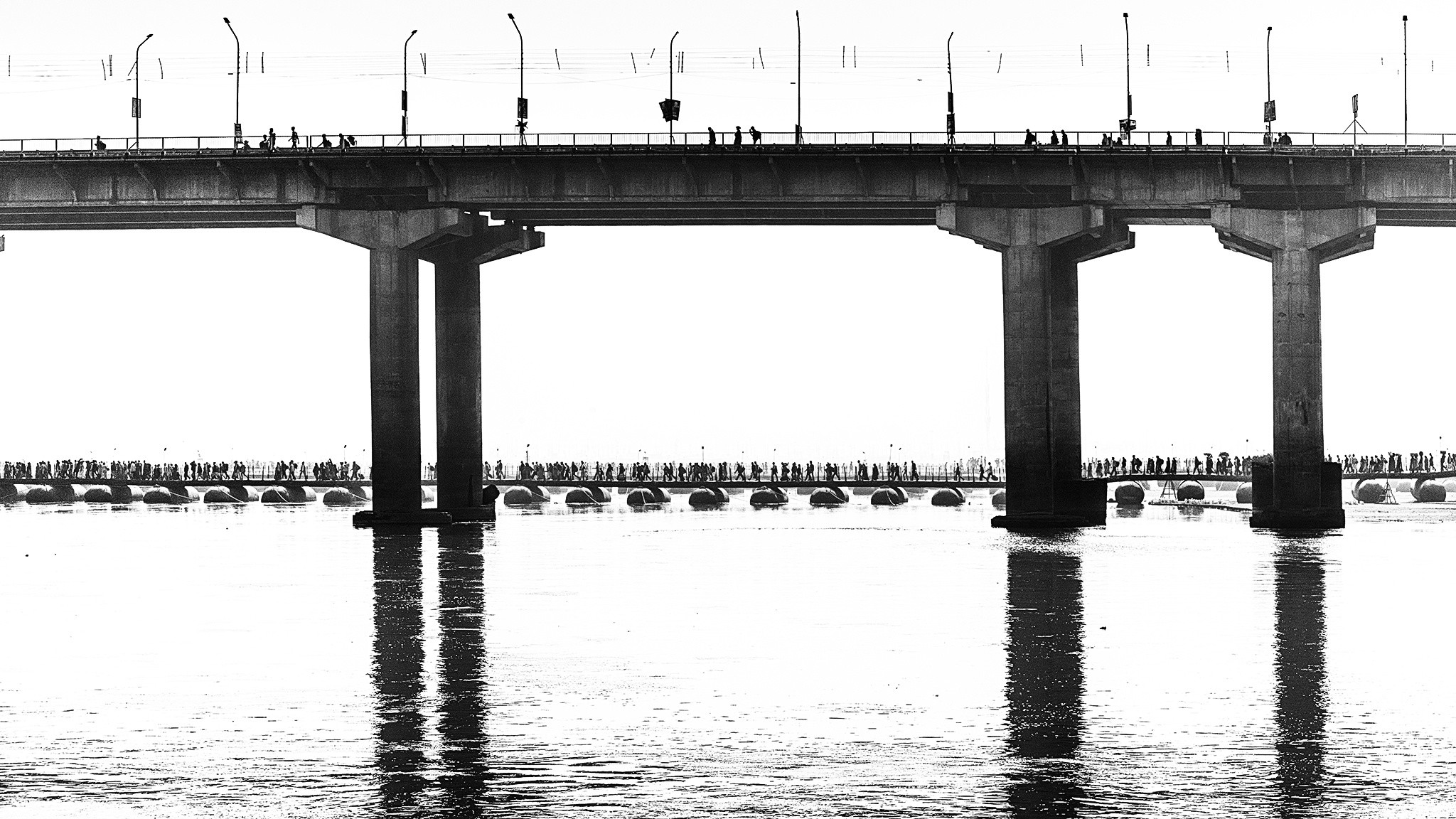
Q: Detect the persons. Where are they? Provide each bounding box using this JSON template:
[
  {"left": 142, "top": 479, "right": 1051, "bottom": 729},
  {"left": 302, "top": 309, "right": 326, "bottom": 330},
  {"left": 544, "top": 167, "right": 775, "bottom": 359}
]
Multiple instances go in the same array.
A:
[
  {"left": 336, "top": 133, "right": 347, "bottom": 148},
  {"left": 288, "top": 126, "right": 300, "bottom": 148},
  {"left": 1262, "top": 132, "right": 1293, "bottom": 146},
  {"left": 1166, "top": 131, "right": 1172, "bottom": 146},
  {"left": 244, "top": 141, "right": 251, "bottom": 149},
  {"left": 733, "top": 126, "right": 742, "bottom": 145},
  {"left": 317, "top": 134, "right": 332, "bottom": 148},
  {"left": 1107, "top": 137, "right": 1114, "bottom": 147},
  {"left": 1051, "top": 130, "right": 1060, "bottom": 145},
  {"left": 707, "top": 127, "right": 717, "bottom": 145},
  {"left": 1101, "top": 133, "right": 1108, "bottom": 146},
  {"left": 0, "top": 450, "right": 1456, "bottom": 482},
  {"left": 259, "top": 128, "right": 276, "bottom": 149},
  {"left": 1024, "top": 129, "right": 1034, "bottom": 146},
  {"left": 95, "top": 135, "right": 106, "bottom": 152},
  {"left": 1060, "top": 129, "right": 1068, "bottom": 146},
  {"left": 749, "top": 126, "right": 761, "bottom": 143},
  {"left": 1116, "top": 137, "right": 1123, "bottom": 146}
]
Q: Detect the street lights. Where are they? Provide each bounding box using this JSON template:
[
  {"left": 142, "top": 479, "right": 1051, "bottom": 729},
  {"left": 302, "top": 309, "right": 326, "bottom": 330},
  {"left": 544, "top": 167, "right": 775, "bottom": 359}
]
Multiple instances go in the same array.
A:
[
  {"left": 224, "top": 16, "right": 241, "bottom": 145},
  {"left": 403, "top": 29, "right": 419, "bottom": 147},
  {"left": 133, "top": 33, "right": 154, "bottom": 148},
  {"left": 507, "top": 13, "right": 525, "bottom": 147},
  {"left": 1400, "top": 14, "right": 1409, "bottom": 145},
  {"left": 1263, "top": 25, "right": 1273, "bottom": 146},
  {"left": 1121, "top": 11, "right": 1133, "bottom": 148}
]
